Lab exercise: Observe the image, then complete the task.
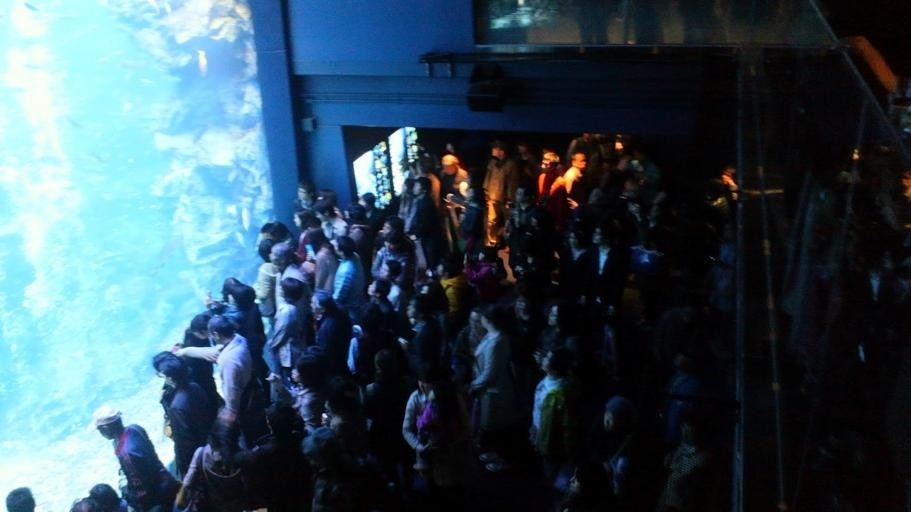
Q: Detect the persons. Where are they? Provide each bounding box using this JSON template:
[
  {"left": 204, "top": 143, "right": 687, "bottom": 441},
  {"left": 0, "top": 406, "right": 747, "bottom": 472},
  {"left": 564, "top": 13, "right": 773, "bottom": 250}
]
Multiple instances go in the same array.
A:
[
  {"left": 71, "top": 483, "right": 129, "bottom": 510},
  {"left": 150, "top": 134, "right": 909, "bottom": 510},
  {"left": 6, "top": 487, "right": 36, "bottom": 510},
  {"left": 88, "top": 405, "right": 181, "bottom": 511}
]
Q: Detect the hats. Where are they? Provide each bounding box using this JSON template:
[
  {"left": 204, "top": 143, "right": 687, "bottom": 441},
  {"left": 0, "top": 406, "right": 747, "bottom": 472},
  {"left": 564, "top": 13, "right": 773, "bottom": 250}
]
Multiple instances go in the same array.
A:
[{"left": 86, "top": 405, "right": 121, "bottom": 432}]
